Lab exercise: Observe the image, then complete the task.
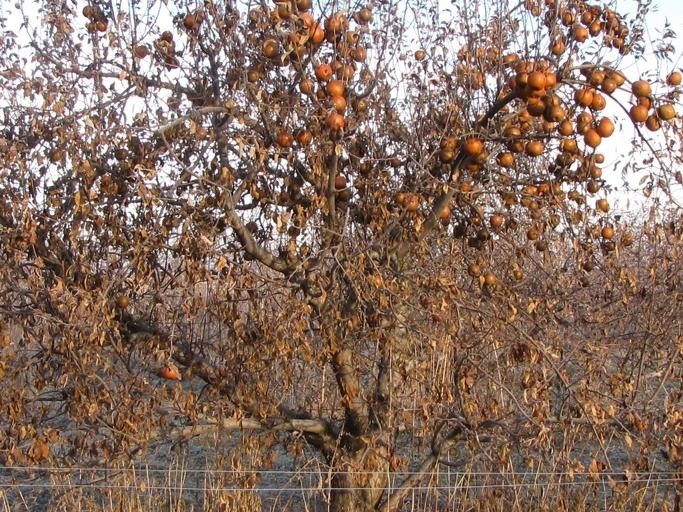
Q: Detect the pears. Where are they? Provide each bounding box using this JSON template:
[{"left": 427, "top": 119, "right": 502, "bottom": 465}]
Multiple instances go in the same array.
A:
[
  {"left": 82, "top": 1, "right": 683, "bottom": 309},
  {"left": 162, "top": 363, "right": 178, "bottom": 380}
]
